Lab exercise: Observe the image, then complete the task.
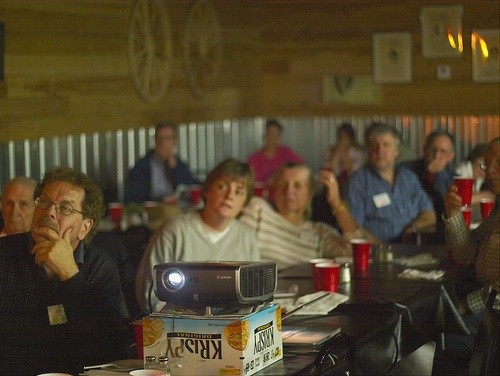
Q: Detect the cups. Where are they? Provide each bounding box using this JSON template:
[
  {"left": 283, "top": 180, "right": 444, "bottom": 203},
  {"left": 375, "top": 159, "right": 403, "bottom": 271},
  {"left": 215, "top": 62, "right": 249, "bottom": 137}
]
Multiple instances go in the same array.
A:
[
  {"left": 133, "top": 319, "right": 143, "bottom": 358},
  {"left": 309, "top": 258, "right": 334, "bottom": 289},
  {"left": 315, "top": 263, "right": 340, "bottom": 292},
  {"left": 461, "top": 208, "right": 472, "bottom": 228},
  {"left": 350, "top": 239, "right": 370, "bottom": 270},
  {"left": 191, "top": 188, "right": 201, "bottom": 204},
  {"left": 480, "top": 200, "right": 494, "bottom": 220},
  {"left": 109, "top": 203, "right": 123, "bottom": 222},
  {"left": 129, "top": 369, "right": 165, "bottom": 376},
  {"left": 452, "top": 177, "right": 474, "bottom": 204},
  {"left": 448, "top": 249, "right": 460, "bottom": 267}
]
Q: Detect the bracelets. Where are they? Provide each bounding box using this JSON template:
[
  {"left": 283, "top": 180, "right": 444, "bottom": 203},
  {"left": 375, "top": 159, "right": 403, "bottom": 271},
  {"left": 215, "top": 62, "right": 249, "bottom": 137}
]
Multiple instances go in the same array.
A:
[
  {"left": 332, "top": 201, "right": 349, "bottom": 215},
  {"left": 412, "top": 222, "right": 417, "bottom": 233}
]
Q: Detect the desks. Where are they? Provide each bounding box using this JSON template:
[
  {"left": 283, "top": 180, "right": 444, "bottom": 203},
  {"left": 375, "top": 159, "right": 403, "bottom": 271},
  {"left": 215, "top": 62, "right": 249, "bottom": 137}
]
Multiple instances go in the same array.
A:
[{"left": 276, "top": 224, "right": 486, "bottom": 376}]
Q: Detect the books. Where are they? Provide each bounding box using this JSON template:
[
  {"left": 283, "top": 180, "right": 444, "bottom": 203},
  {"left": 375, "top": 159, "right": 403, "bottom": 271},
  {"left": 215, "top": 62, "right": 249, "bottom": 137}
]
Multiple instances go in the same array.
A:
[{"left": 282, "top": 325, "right": 343, "bottom": 346}]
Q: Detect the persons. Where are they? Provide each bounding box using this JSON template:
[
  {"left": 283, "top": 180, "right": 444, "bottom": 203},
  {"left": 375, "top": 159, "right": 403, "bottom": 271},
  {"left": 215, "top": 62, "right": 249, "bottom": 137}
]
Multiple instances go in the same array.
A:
[
  {"left": 134, "top": 157, "right": 261, "bottom": 315},
  {"left": 237, "top": 161, "right": 367, "bottom": 273},
  {"left": 310, "top": 122, "right": 366, "bottom": 236},
  {"left": 1, "top": 166, "right": 138, "bottom": 376},
  {"left": 346, "top": 123, "right": 437, "bottom": 244},
  {"left": 126, "top": 120, "right": 201, "bottom": 205},
  {"left": 452, "top": 144, "right": 497, "bottom": 207},
  {"left": 246, "top": 119, "right": 307, "bottom": 200},
  {"left": 398, "top": 131, "right": 462, "bottom": 244},
  {"left": 432, "top": 137, "right": 500, "bottom": 376},
  {"left": 0, "top": 176, "right": 39, "bottom": 237}
]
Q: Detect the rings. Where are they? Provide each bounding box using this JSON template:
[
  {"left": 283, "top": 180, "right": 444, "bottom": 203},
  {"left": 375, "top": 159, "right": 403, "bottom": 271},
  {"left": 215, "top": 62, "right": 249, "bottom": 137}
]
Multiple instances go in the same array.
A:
[{"left": 330, "top": 177, "right": 335, "bottom": 184}]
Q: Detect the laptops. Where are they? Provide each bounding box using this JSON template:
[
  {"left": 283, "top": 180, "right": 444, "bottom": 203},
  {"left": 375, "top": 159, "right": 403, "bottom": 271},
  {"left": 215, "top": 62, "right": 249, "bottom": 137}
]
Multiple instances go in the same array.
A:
[{"left": 281, "top": 293, "right": 342, "bottom": 346}]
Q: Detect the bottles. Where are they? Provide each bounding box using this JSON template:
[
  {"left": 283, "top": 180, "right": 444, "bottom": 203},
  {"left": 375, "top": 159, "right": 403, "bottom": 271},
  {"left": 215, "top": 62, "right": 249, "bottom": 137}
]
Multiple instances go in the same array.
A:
[
  {"left": 340, "top": 263, "right": 351, "bottom": 283},
  {"left": 145, "top": 356, "right": 158, "bottom": 370},
  {"left": 373, "top": 244, "right": 393, "bottom": 263},
  {"left": 156, "top": 357, "right": 170, "bottom": 376}
]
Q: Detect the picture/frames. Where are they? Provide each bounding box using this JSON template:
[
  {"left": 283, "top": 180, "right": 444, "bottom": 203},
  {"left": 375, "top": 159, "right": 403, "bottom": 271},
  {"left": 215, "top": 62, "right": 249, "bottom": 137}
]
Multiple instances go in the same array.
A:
[
  {"left": 421, "top": 5, "right": 464, "bottom": 59},
  {"left": 371, "top": 30, "right": 413, "bottom": 85},
  {"left": 471, "top": 29, "right": 500, "bottom": 84}
]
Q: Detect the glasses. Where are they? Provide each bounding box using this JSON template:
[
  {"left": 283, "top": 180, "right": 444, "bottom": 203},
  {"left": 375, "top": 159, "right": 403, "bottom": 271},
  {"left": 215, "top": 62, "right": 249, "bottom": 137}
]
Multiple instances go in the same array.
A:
[{"left": 34, "top": 197, "right": 86, "bottom": 216}]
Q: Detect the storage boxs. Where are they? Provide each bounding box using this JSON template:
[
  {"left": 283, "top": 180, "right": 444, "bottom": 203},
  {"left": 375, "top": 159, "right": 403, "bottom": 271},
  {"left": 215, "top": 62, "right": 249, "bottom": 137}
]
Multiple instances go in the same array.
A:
[{"left": 143, "top": 303, "right": 284, "bottom": 376}]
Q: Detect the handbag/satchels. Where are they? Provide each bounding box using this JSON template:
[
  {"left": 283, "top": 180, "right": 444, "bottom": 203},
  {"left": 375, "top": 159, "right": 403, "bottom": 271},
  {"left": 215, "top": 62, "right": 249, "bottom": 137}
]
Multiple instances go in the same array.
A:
[{"left": 313, "top": 335, "right": 356, "bottom": 376}]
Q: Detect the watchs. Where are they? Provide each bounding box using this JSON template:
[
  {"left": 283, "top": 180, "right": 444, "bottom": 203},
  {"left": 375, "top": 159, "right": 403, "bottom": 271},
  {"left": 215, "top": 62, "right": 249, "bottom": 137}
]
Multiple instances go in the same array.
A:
[{"left": 458, "top": 301, "right": 467, "bottom": 316}]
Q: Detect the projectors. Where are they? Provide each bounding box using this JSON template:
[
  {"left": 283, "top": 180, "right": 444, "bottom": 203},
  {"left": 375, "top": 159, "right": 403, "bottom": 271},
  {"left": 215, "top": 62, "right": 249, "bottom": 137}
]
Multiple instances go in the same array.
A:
[{"left": 152, "top": 260, "right": 278, "bottom": 306}]
